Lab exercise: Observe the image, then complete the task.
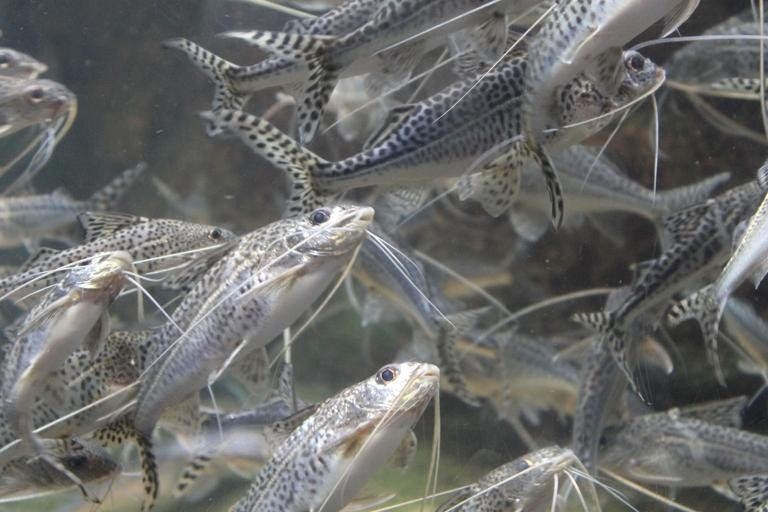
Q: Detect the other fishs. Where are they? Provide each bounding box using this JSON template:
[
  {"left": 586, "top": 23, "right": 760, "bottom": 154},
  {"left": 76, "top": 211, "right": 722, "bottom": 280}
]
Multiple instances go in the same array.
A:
[{"left": 0, "top": 0, "right": 768, "bottom": 511}]
